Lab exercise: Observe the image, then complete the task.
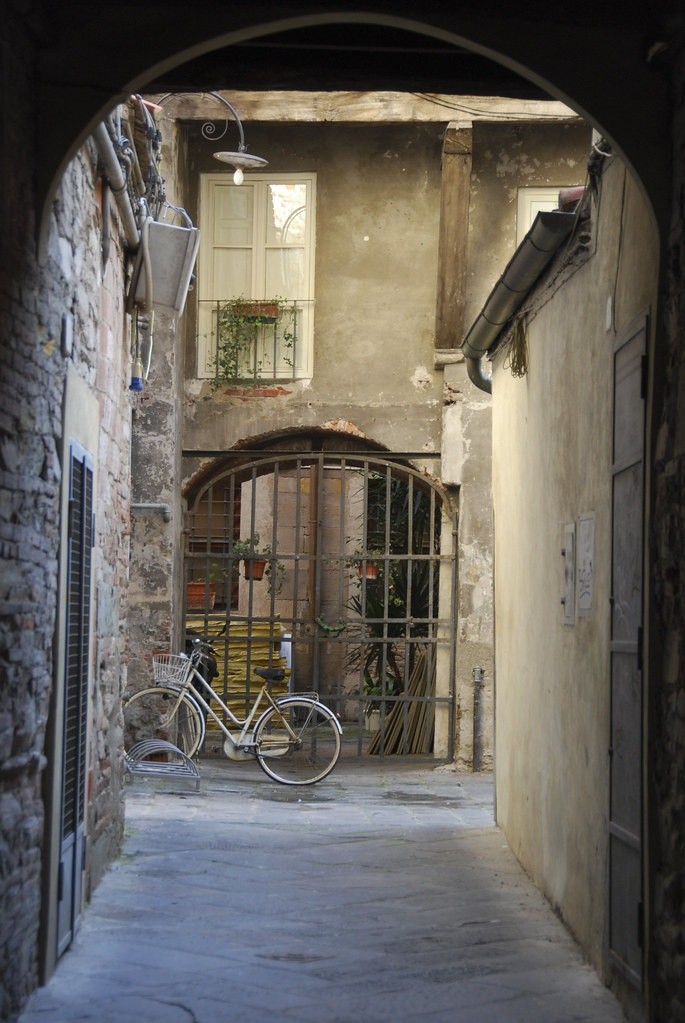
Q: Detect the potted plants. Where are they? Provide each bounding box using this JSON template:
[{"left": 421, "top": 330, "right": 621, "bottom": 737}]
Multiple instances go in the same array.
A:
[
  {"left": 336, "top": 543, "right": 405, "bottom": 607},
  {"left": 234, "top": 529, "right": 287, "bottom": 596},
  {"left": 202, "top": 293, "right": 298, "bottom": 401},
  {"left": 354, "top": 681, "right": 380, "bottom": 731},
  {"left": 187, "top": 563, "right": 228, "bottom": 610}
]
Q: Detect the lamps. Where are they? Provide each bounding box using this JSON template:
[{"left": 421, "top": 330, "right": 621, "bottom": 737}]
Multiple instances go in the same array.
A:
[{"left": 156, "top": 93, "right": 269, "bottom": 185}]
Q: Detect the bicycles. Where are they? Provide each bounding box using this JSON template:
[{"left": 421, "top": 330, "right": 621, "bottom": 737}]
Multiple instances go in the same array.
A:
[{"left": 119, "top": 636, "right": 345, "bottom": 787}]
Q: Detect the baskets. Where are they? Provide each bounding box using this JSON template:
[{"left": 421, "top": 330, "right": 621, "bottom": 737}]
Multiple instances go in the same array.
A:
[{"left": 152, "top": 654, "right": 191, "bottom": 684}]
